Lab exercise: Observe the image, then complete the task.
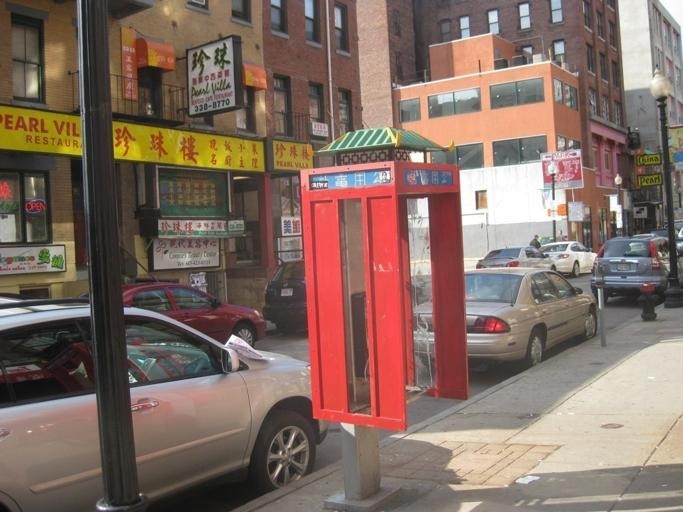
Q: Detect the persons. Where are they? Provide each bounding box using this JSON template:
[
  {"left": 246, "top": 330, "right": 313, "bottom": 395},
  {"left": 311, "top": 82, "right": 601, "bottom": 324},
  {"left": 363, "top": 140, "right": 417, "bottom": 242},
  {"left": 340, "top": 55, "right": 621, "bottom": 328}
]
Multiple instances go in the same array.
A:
[{"left": 529, "top": 235, "right": 541, "bottom": 249}]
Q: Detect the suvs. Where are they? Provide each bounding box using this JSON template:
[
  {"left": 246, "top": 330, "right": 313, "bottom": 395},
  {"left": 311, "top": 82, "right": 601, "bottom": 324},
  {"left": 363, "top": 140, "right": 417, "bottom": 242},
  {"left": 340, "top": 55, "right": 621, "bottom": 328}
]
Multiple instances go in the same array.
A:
[
  {"left": 261, "top": 259, "right": 308, "bottom": 337},
  {"left": 0, "top": 292, "right": 329, "bottom": 512}
]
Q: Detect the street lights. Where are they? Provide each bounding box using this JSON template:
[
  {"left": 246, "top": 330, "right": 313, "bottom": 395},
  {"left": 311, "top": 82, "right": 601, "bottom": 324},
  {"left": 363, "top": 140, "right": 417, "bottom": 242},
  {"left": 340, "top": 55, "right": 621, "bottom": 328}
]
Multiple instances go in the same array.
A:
[
  {"left": 546, "top": 162, "right": 558, "bottom": 241},
  {"left": 612, "top": 172, "right": 626, "bottom": 237},
  {"left": 647, "top": 62, "right": 683, "bottom": 308}
]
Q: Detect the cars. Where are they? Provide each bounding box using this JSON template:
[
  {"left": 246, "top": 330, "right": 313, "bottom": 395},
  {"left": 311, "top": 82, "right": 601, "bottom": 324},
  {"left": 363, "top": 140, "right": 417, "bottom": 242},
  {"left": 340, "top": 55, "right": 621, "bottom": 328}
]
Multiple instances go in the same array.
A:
[
  {"left": 588, "top": 216, "right": 682, "bottom": 306},
  {"left": 538, "top": 238, "right": 598, "bottom": 278},
  {"left": 408, "top": 268, "right": 599, "bottom": 365},
  {"left": 79, "top": 278, "right": 265, "bottom": 368},
  {"left": 476, "top": 245, "right": 558, "bottom": 274}
]
